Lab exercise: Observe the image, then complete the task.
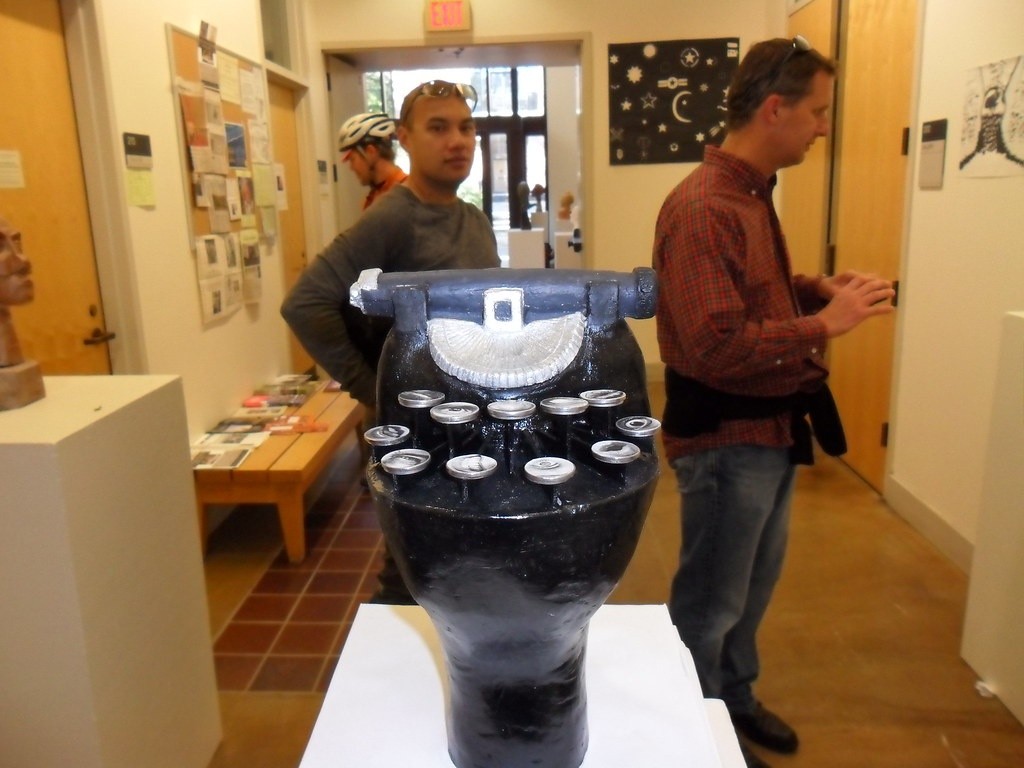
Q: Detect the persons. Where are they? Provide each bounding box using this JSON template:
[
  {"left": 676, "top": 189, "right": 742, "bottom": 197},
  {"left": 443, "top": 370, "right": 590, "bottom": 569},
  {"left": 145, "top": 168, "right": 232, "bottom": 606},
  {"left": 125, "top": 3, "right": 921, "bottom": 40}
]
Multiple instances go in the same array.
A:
[
  {"left": 0, "top": 217, "right": 37, "bottom": 366},
  {"left": 279, "top": 80, "right": 501, "bottom": 606},
  {"left": 336, "top": 110, "right": 407, "bottom": 209},
  {"left": 653, "top": 39, "right": 896, "bottom": 768}
]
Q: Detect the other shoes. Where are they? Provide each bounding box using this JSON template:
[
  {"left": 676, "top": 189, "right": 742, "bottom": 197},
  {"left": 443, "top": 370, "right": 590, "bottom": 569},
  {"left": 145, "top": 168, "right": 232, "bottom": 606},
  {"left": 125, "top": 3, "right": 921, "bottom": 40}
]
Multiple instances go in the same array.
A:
[
  {"left": 744, "top": 750, "right": 768, "bottom": 767},
  {"left": 731, "top": 703, "right": 797, "bottom": 753}
]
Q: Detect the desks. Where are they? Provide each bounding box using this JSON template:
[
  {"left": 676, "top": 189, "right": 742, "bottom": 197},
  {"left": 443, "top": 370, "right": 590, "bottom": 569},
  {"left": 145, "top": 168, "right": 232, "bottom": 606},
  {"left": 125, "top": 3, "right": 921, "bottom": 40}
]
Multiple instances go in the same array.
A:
[{"left": 192, "top": 378, "right": 370, "bottom": 565}]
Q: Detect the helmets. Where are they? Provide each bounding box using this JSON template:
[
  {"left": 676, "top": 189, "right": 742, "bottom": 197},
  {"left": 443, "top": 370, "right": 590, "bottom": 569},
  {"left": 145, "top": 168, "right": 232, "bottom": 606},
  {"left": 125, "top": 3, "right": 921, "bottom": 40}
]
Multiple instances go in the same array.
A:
[{"left": 337, "top": 112, "right": 400, "bottom": 152}]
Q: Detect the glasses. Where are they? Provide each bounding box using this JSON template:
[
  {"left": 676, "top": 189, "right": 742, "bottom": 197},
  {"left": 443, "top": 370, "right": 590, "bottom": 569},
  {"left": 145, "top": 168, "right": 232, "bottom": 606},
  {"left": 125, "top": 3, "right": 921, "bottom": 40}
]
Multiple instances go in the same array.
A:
[
  {"left": 771, "top": 35, "right": 812, "bottom": 78},
  {"left": 403, "top": 80, "right": 478, "bottom": 123}
]
